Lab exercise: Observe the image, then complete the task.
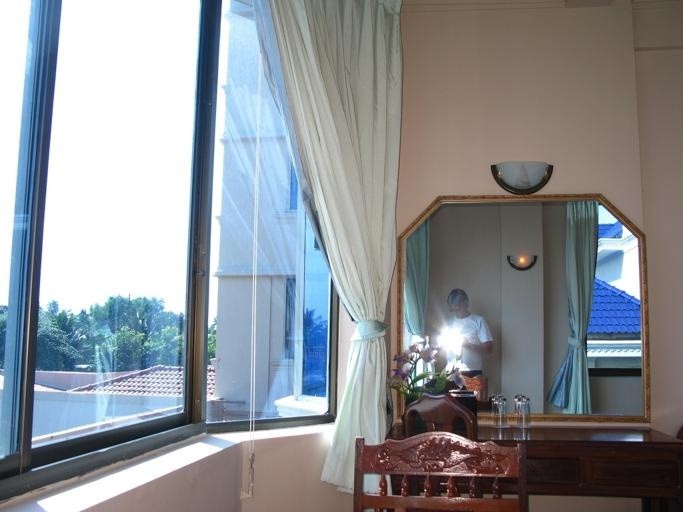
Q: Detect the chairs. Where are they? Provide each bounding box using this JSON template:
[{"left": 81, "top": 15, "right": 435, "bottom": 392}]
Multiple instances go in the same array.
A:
[
  {"left": 403, "top": 392, "right": 477, "bottom": 495},
  {"left": 347, "top": 434, "right": 529, "bottom": 512}
]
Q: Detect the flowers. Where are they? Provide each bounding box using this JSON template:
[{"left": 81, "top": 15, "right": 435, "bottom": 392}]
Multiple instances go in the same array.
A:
[{"left": 385, "top": 343, "right": 466, "bottom": 409}]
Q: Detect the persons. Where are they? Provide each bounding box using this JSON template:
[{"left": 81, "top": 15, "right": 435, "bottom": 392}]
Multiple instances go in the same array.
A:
[{"left": 432, "top": 289, "right": 494, "bottom": 390}]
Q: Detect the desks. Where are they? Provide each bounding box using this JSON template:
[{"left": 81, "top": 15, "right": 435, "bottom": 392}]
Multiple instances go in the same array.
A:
[{"left": 383, "top": 421, "right": 683, "bottom": 512}]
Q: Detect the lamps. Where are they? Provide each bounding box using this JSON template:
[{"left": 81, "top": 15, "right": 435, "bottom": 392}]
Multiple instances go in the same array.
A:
[
  {"left": 507, "top": 255, "right": 537, "bottom": 270},
  {"left": 491, "top": 161, "right": 553, "bottom": 195}
]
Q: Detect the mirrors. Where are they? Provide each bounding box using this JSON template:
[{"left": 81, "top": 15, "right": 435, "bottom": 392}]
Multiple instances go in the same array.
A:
[{"left": 396, "top": 193, "right": 651, "bottom": 424}]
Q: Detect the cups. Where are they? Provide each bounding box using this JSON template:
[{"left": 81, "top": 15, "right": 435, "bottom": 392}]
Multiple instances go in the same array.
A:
[{"left": 490, "top": 393, "right": 534, "bottom": 430}]
